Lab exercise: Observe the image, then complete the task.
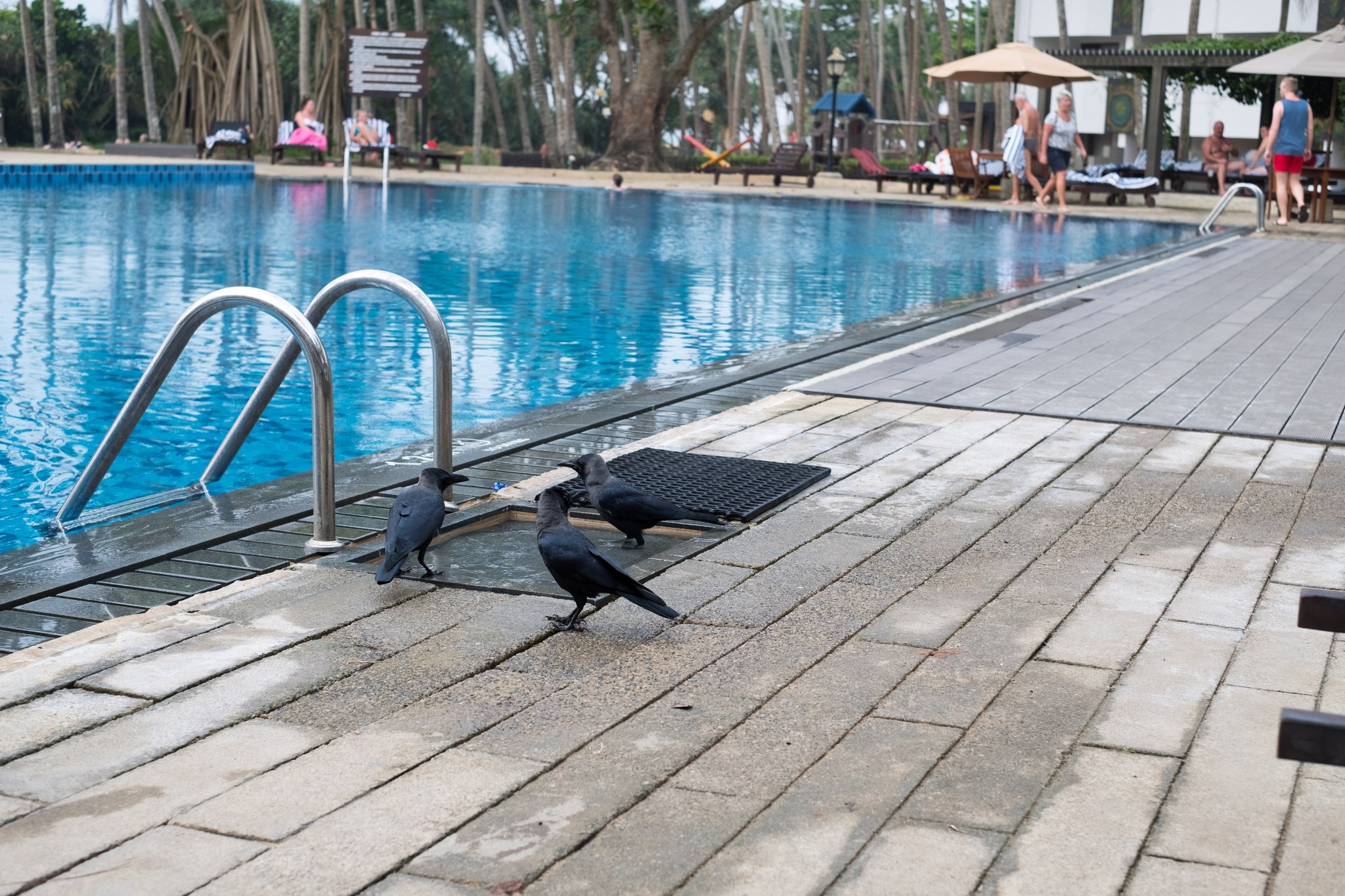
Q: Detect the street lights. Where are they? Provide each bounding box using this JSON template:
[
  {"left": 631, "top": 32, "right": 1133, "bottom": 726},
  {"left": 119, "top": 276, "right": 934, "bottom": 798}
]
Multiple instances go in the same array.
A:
[
  {"left": 824, "top": 47, "right": 847, "bottom": 171},
  {"left": 592, "top": 88, "right": 605, "bottom": 164}
]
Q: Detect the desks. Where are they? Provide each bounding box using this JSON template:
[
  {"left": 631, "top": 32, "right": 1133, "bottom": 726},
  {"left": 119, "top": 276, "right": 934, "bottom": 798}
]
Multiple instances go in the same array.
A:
[{"left": 1301, "top": 166, "right": 1345, "bottom": 224}]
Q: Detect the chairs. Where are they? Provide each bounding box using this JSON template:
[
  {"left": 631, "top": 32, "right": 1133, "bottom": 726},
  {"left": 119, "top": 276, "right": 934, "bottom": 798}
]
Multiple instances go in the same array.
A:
[
  {"left": 343, "top": 117, "right": 464, "bottom": 171},
  {"left": 195, "top": 121, "right": 252, "bottom": 161},
  {"left": 269, "top": 121, "right": 326, "bottom": 166},
  {"left": 1268, "top": 163, "right": 1293, "bottom": 222},
  {"left": 709, "top": 142, "right": 817, "bottom": 188},
  {"left": 852, "top": 147, "right": 1051, "bottom": 200},
  {"left": 1061, "top": 149, "right": 1333, "bottom": 208}
]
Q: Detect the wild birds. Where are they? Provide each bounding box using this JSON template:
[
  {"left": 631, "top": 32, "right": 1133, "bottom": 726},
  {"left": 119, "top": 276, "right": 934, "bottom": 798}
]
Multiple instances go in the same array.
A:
[
  {"left": 375, "top": 467, "right": 470, "bottom": 586},
  {"left": 536, "top": 485, "right": 682, "bottom": 632},
  {"left": 557, "top": 453, "right": 729, "bottom": 549}
]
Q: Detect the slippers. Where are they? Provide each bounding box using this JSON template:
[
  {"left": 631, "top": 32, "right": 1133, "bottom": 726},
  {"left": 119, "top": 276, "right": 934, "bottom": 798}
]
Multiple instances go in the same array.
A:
[
  {"left": 1273, "top": 220, "right": 1288, "bottom": 226},
  {"left": 1297, "top": 206, "right": 1309, "bottom": 222}
]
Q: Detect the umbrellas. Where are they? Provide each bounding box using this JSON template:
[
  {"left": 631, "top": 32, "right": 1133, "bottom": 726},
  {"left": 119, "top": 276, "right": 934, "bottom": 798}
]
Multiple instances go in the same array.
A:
[
  {"left": 922, "top": 43, "right": 1101, "bottom": 126},
  {"left": 1225, "top": 17, "right": 1345, "bottom": 169}
]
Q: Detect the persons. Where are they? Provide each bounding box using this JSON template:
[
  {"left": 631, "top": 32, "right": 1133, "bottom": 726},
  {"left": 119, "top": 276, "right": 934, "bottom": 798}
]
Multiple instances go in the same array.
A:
[
  {"left": 1264, "top": 77, "right": 1312, "bottom": 225},
  {"left": 350, "top": 110, "right": 383, "bottom": 167},
  {"left": 1201, "top": 122, "right": 1273, "bottom": 196},
  {"left": 1036, "top": 90, "right": 1086, "bottom": 213},
  {"left": 289, "top": 98, "right": 327, "bottom": 149},
  {"left": 1000, "top": 93, "right": 1050, "bottom": 205}
]
